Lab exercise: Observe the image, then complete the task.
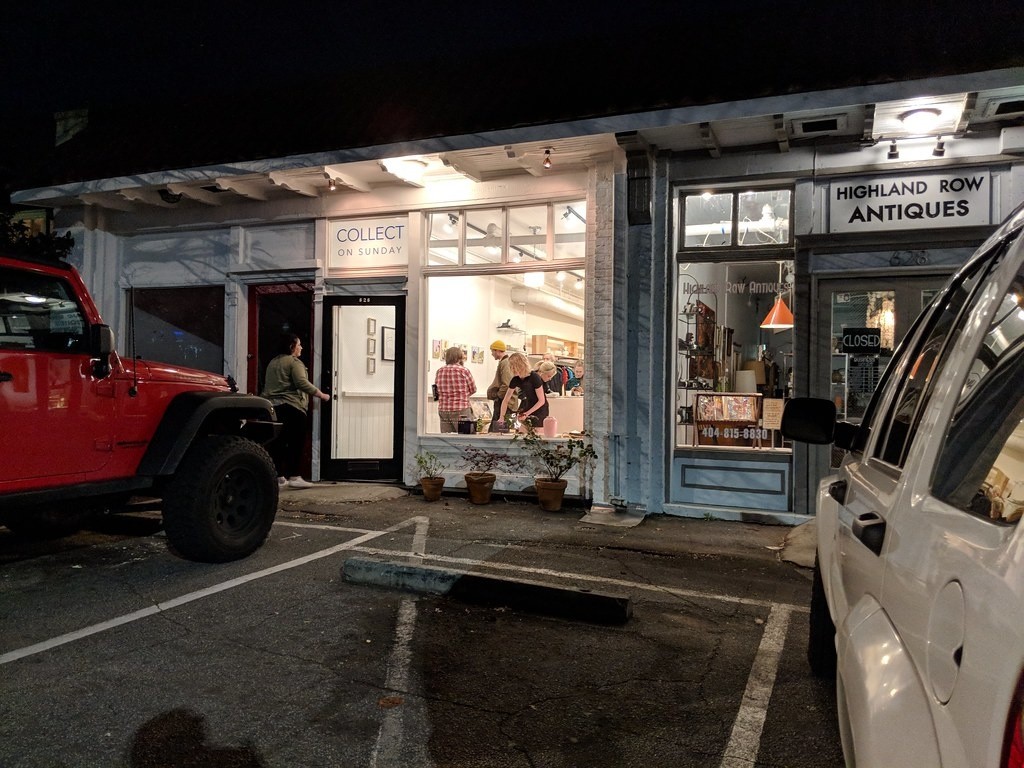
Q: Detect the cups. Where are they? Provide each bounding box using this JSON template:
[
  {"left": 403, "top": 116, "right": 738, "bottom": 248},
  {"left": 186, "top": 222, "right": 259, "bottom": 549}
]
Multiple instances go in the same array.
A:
[{"left": 834, "top": 386, "right": 842, "bottom": 413}]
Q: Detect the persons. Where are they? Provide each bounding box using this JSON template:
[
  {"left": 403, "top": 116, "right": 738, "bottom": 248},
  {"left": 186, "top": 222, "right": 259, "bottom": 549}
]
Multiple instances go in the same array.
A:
[
  {"left": 260, "top": 336, "right": 330, "bottom": 487},
  {"left": 435, "top": 347, "right": 478, "bottom": 433},
  {"left": 543, "top": 352, "right": 563, "bottom": 395},
  {"left": 571, "top": 359, "right": 585, "bottom": 392},
  {"left": 533, "top": 361, "right": 557, "bottom": 394},
  {"left": 498, "top": 353, "right": 549, "bottom": 427},
  {"left": 487, "top": 340, "right": 519, "bottom": 433}
]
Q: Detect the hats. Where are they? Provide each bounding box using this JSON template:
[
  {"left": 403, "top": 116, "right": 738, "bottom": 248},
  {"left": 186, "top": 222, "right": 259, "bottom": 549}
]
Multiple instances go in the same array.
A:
[{"left": 490, "top": 340, "right": 505, "bottom": 351}]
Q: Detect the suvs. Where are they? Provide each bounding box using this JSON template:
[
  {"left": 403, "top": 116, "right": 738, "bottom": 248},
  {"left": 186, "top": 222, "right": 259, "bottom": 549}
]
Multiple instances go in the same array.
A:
[
  {"left": 0, "top": 251, "right": 284, "bottom": 563},
  {"left": 780, "top": 200, "right": 1024, "bottom": 768}
]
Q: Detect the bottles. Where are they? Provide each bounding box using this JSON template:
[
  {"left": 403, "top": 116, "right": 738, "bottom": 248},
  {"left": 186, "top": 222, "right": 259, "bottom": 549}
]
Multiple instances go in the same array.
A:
[
  {"left": 459, "top": 415, "right": 470, "bottom": 421},
  {"left": 573, "top": 385, "right": 581, "bottom": 396},
  {"left": 543, "top": 417, "right": 558, "bottom": 438}
]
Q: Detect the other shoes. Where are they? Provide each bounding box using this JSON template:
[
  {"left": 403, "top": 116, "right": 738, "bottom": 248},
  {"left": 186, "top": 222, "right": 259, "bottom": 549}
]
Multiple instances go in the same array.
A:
[
  {"left": 288, "top": 476, "right": 314, "bottom": 488},
  {"left": 277, "top": 476, "right": 288, "bottom": 486}
]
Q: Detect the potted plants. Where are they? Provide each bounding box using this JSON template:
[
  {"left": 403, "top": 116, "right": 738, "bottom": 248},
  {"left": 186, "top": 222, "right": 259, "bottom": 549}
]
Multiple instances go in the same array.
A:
[{"left": 413, "top": 414, "right": 599, "bottom": 512}]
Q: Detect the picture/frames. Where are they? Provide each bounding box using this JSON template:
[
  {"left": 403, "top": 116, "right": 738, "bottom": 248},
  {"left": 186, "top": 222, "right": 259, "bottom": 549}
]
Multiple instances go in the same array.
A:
[
  {"left": 367, "top": 317, "right": 376, "bottom": 374},
  {"left": 381, "top": 326, "right": 395, "bottom": 361}
]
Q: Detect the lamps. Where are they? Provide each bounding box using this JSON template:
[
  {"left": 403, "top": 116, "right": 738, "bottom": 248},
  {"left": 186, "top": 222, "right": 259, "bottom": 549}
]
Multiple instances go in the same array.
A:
[
  {"left": 328, "top": 179, "right": 336, "bottom": 191},
  {"left": 443, "top": 216, "right": 456, "bottom": 234},
  {"left": 523, "top": 226, "right": 544, "bottom": 287},
  {"left": 899, "top": 108, "right": 941, "bottom": 134},
  {"left": 888, "top": 138, "right": 900, "bottom": 159},
  {"left": 542, "top": 150, "right": 551, "bottom": 170},
  {"left": 932, "top": 134, "right": 945, "bottom": 156},
  {"left": 760, "top": 261, "right": 794, "bottom": 328}
]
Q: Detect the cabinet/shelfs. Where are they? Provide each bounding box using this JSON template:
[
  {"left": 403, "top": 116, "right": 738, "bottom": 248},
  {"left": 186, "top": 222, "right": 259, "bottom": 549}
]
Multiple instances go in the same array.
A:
[
  {"left": 496, "top": 327, "right": 527, "bottom": 355},
  {"left": 675, "top": 273, "right": 718, "bottom": 444},
  {"left": 780, "top": 351, "right": 848, "bottom": 448}
]
